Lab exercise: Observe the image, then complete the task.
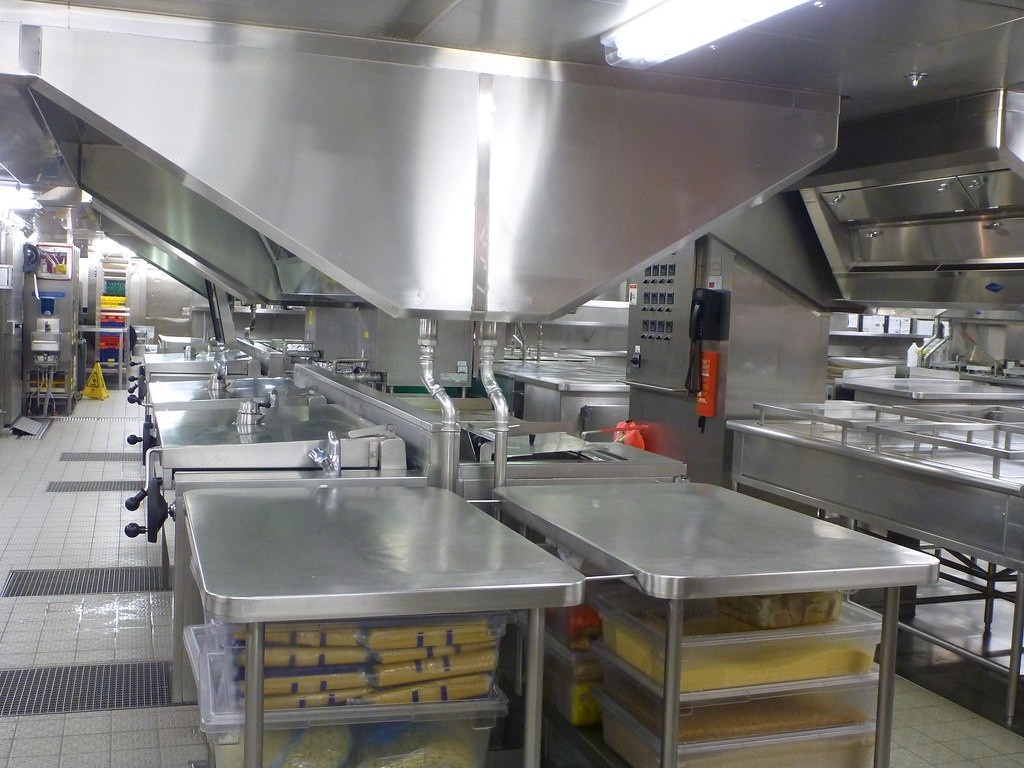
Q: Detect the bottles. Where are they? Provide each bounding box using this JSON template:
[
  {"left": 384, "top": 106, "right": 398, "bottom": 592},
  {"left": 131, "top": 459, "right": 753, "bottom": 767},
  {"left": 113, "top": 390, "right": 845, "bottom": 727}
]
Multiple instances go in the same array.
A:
[{"left": 907, "top": 343, "right": 918, "bottom": 367}]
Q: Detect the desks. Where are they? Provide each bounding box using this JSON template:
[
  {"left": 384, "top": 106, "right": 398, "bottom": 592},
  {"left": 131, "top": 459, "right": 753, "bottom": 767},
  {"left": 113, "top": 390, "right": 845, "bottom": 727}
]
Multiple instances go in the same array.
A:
[
  {"left": 168, "top": 485, "right": 587, "bottom": 765},
  {"left": 489, "top": 479, "right": 940, "bottom": 768}
]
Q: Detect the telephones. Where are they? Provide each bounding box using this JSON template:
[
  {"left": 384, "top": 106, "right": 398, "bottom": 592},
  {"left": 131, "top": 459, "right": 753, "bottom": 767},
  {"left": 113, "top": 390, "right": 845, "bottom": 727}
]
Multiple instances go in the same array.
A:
[{"left": 689, "top": 288, "right": 725, "bottom": 343}]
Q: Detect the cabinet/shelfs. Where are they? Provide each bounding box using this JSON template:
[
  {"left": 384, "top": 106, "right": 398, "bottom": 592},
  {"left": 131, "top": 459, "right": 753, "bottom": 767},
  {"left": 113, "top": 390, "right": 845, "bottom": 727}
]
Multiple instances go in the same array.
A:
[{"left": 94, "top": 255, "right": 131, "bottom": 381}]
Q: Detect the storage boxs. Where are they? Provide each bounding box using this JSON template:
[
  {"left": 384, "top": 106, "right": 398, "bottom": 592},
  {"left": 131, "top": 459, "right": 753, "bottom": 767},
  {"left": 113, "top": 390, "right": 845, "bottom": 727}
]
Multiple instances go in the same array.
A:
[
  {"left": 591, "top": 590, "right": 885, "bottom": 696},
  {"left": 513, "top": 609, "right": 612, "bottom": 727},
  {"left": 220, "top": 609, "right": 513, "bottom": 713},
  {"left": 193, "top": 645, "right": 510, "bottom": 768},
  {"left": 595, "top": 684, "right": 877, "bottom": 768},
  {"left": 181, "top": 624, "right": 246, "bottom": 690}
]
